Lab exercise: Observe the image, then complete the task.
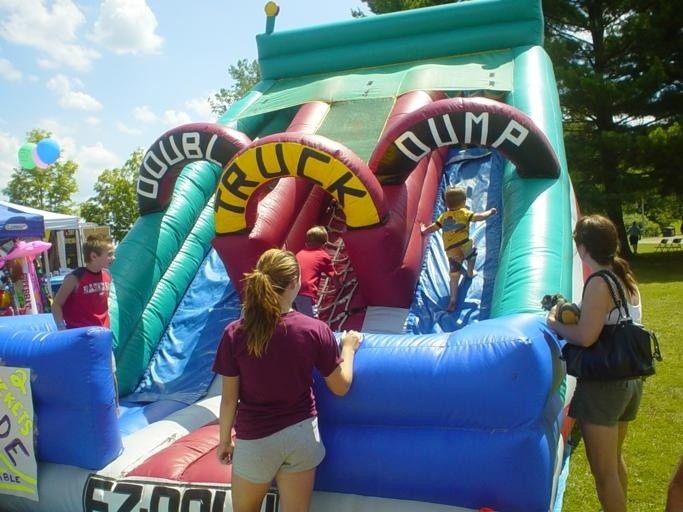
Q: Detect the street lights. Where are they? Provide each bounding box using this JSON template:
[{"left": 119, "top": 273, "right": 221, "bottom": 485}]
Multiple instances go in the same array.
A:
[{"left": 558, "top": 269, "right": 662, "bottom": 381}]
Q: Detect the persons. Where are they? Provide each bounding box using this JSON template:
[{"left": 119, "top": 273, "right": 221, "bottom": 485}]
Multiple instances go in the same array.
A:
[
  {"left": 292, "top": 225, "right": 343, "bottom": 318},
  {"left": 212, "top": 248, "right": 365, "bottom": 512},
  {"left": 548, "top": 214, "right": 647, "bottom": 512},
  {"left": 626, "top": 221, "right": 642, "bottom": 255},
  {"left": 420, "top": 186, "right": 498, "bottom": 313},
  {"left": 49, "top": 232, "right": 122, "bottom": 419}
]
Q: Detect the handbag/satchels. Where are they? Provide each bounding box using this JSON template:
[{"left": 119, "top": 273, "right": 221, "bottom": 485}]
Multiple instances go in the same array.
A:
[{"left": 56, "top": 322, "right": 65, "bottom": 328}]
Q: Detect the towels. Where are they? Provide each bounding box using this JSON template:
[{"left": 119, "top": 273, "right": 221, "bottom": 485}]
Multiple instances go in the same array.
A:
[{"left": 663, "top": 227, "right": 674, "bottom": 236}]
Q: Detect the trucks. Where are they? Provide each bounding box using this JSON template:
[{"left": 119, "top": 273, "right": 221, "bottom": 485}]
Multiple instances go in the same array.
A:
[
  {"left": 36, "top": 138, "right": 61, "bottom": 164},
  {"left": 33, "top": 145, "right": 49, "bottom": 170},
  {"left": 18, "top": 143, "right": 36, "bottom": 170}
]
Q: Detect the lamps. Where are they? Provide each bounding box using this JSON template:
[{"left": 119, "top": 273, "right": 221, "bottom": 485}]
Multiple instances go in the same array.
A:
[
  {"left": 653, "top": 238, "right": 668, "bottom": 256},
  {"left": 667, "top": 239, "right": 682, "bottom": 256}
]
